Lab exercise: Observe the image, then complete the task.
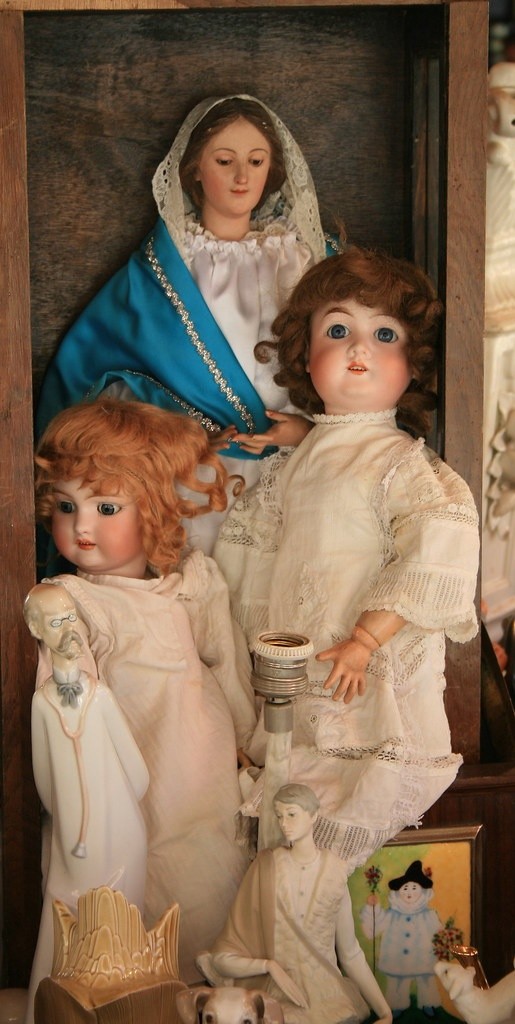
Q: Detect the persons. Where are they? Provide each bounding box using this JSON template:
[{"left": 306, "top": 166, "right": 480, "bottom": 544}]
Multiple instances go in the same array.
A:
[
  {"left": 35, "top": 398, "right": 257, "bottom": 988},
  {"left": 39, "top": 92, "right": 348, "bottom": 556},
  {"left": 435, "top": 962, "right": 515, "bottom": 1024},
  {"left": 210, "top": 784, "right": 393, "bottom": 1024},
  {"left": 24, "top": 583, "right": 149, "bottom": 1024},
  {"left": 212, "top": 242, "right": 482, "bottom": 877}
]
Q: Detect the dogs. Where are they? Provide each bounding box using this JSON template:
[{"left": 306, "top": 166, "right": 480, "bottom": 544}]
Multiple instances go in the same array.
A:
[
  {"left": 433, "top": 957, "right": 515, "bottom": 1024},
  {"left": 175, "top": 986, "right": 284, "bottom": 1024}
]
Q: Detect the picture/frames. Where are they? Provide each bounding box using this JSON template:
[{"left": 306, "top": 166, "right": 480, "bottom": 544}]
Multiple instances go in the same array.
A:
[{"left": 331, "top": 821, "right": 489, "bottom": 1018}]
